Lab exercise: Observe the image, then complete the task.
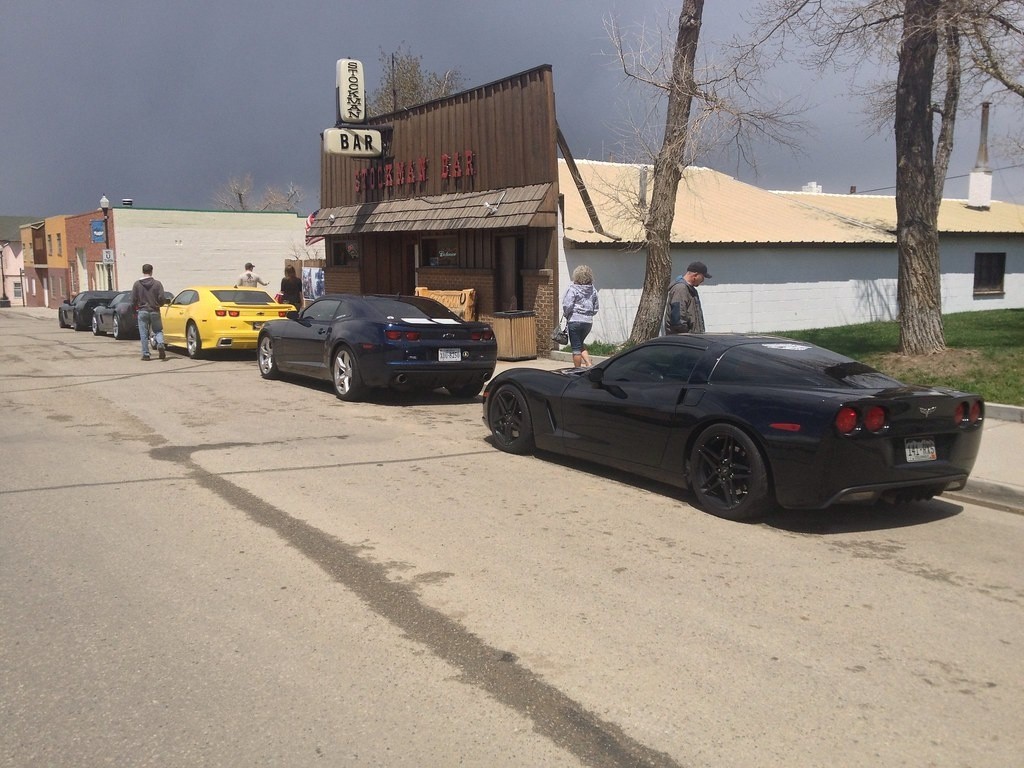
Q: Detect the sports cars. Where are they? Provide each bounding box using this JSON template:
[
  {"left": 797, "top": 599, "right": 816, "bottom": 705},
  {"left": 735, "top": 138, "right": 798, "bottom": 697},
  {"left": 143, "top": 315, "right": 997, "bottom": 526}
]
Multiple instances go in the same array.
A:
[
  {"left": 57, "top": 289, "right": 123, "bottom": 332},
  {"left": 256, "top": 293, "right": 498, "bottom": 402},
  {"left": 89, "top": 290, "right": 178, "bottom": 342},
  {"left": 478, "top": 329, "right": 987, "bottom": 524},
  {"left": 149, "top": 284, "right": 298, "bottom": 360}
]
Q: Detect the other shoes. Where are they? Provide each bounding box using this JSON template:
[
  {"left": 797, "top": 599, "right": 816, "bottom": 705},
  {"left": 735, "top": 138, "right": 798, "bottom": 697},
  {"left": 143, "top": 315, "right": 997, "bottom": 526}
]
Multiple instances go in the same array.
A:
[
  {"left": 157, "top": 344, "right": 166, "bottom": 359},
  {"left": 140, "top": 355, "right": 150, "bottom": 360}
]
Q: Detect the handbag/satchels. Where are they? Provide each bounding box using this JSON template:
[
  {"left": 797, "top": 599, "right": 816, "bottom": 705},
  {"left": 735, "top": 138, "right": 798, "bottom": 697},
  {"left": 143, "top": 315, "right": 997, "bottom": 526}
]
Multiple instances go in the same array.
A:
[
  {"left": 550, "top": 316, "right": 569, "bottom": 345},
  {"left": 274, "top": 290, "right": 284, "bottom": 304}
]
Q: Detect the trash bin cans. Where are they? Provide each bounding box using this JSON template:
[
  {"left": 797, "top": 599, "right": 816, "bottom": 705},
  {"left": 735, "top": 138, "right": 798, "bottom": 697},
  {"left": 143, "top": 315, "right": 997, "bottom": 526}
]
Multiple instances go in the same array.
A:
[{"left": 492, "top": 308, "right": 538, "bottom": 364}]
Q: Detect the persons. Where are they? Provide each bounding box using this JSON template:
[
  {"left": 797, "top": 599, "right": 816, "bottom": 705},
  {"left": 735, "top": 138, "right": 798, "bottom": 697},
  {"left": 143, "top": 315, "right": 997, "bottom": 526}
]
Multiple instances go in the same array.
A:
[
  {"left": 281, "top": 265, "right": 305, "bottom": 311},
  {"left": 665, "top": 262, "right": 712, "bottom": 335},
  {"left": 563, "top": 265, "right": 599, "bottom": 368},
  {"left": 235, "top": 264, "right": 271, "bottom": 288},
  {"left": 131, "top": 264, "right": 166, "bottom": 360}
]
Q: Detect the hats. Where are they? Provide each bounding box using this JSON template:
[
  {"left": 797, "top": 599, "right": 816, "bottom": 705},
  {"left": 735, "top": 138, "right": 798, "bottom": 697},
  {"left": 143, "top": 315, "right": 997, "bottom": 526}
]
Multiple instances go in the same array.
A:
[
  {"left": 687, "top": 261, "right": 712, "bottom": 278},
  {"left": 244, "top": 262, "right": 255, "bottom": 267}
]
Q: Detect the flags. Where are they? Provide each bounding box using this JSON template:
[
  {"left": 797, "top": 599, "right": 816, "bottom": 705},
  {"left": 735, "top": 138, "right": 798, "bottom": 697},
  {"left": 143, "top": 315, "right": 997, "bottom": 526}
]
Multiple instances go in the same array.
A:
[{"left": 305, "top": 208, "right": 325, "bottom": 246}]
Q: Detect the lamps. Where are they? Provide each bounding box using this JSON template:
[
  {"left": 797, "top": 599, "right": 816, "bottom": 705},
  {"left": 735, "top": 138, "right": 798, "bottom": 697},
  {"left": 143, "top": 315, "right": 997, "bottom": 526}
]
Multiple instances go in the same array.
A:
[
  {"left": 328, "top": 214, "right": 335, "bottom": 223},
  {"left": 484, "top": 203, "right": 498, "bottom": 214}
]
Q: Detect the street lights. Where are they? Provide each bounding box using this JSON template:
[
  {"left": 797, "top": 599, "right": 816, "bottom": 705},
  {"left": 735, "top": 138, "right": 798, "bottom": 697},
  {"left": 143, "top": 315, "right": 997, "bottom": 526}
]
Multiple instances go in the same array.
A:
[
  {"left": 0, "top": 244, "right": 11, "bottom": 307},
  {"left": 98, "top": 194, "right": 113, "bottom": 290}
]
